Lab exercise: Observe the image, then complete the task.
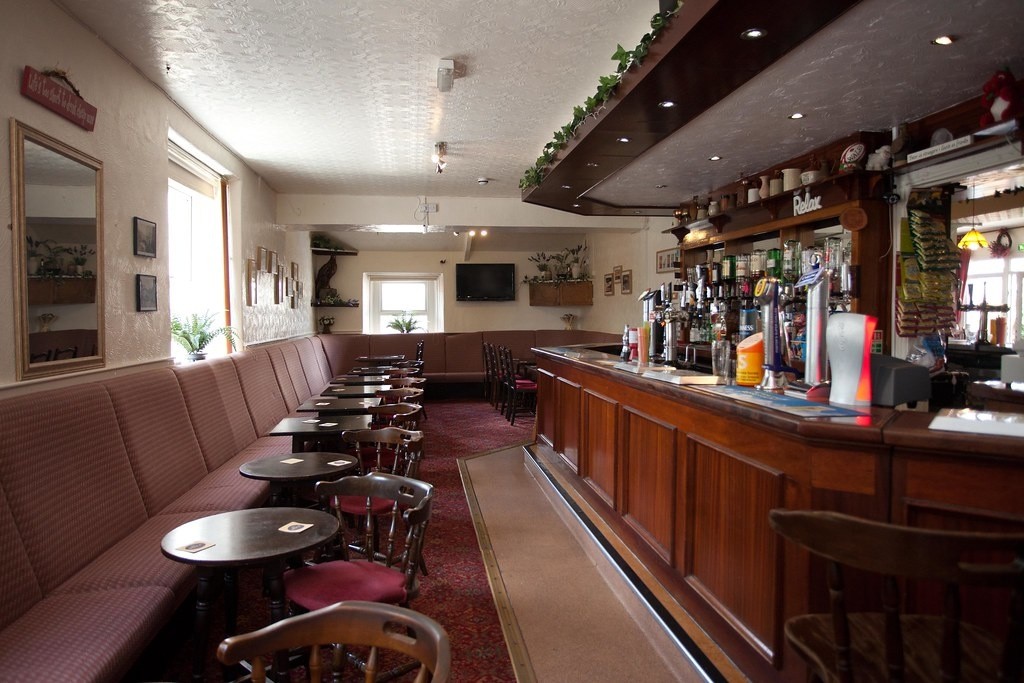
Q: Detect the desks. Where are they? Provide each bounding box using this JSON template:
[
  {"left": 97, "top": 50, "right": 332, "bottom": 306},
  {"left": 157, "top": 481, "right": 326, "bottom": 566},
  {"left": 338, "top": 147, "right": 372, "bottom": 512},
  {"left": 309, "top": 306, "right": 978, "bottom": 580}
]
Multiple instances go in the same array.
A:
[
  {"left": 354, "top": 355, "right": 405, "bottom": 366},
  {"left": 162, "top": 508, "right": 341, "bottom": 683},
  {"left": 346, "top": 366, "right": 398, "bottom": 375},
  {"left": 269, "top": 415, "right": 372, "bottom": 453},
  {"left": 319, "top": 386, "right": 392, "bottom": 396},
  {"left": 238, "top": 452, "right": 358, "bottom": 508},
  {"left": 330, "top": 375, "right": 391, "bottom": 386},
  {"left": 296, "top": 397, "right": 382, "bottom": 414}
]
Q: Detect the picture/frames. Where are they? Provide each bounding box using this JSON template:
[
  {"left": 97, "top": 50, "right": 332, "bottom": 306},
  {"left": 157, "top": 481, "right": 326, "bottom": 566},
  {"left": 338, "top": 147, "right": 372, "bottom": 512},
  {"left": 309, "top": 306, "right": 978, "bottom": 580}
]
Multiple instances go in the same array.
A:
[
  {"left": 135, "top": 275, "right": 158, "bottom": 312},
  {"left": 603, "top": 265, "right": 632, "bottom": 296},
  {"left": 249, "top": 247, "right": 303, "bottom": 309},
  {"left": 656, "top": 247, "right": 680, "bottom": 273},
  {"left": 132, "top": 216, "right": 156, "bottom": 258}
]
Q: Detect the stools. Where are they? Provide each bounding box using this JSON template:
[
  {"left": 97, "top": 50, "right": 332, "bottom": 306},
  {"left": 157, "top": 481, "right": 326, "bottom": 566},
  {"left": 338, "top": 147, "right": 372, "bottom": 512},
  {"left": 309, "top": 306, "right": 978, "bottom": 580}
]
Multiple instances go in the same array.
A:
[{"left": 769, "top": 508, "right": 1024, "bottom": 683}]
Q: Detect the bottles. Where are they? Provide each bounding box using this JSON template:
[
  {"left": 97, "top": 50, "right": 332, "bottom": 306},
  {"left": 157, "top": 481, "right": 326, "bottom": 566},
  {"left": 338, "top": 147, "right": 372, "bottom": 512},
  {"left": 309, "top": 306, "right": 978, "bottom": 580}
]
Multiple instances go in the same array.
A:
[
  {"left": 758, "top": 176, "right": 771, "bottom": 199},
  {"left": 638, "top": 236, "right": 851, "bottom": 347},
  {"left": 708, "top": 194, "right": 736, "bottom": 216},
  {"left": 620, "top": 324, "right": 631, "bottom": 362}
]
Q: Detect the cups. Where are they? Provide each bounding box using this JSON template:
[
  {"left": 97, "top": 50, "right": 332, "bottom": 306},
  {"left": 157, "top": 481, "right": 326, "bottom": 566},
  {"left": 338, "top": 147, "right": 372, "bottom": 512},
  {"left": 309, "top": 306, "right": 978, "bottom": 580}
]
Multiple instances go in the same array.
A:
[
  {"left": 782, "top": 168, "right": 801, "bottom": 191},
  {"left": 711, "top": 339, "right": 730, "bottom": 385},
  {"left": 769, "top": 179, "right": 783, "bottom": 197},
  {"left": 748, "top": 189, "right": 759, "bottom": 204}
]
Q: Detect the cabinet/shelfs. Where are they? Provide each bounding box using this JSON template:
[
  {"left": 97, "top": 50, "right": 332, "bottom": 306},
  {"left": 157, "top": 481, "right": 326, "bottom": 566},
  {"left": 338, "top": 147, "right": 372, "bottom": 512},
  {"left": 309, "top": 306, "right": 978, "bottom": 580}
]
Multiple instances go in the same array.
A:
[
  {"left": 309, "top": 246, "right": 357, "bottom": 334},
  {"left": 661, "top": 131, "right": 892, "bottom": 374}
]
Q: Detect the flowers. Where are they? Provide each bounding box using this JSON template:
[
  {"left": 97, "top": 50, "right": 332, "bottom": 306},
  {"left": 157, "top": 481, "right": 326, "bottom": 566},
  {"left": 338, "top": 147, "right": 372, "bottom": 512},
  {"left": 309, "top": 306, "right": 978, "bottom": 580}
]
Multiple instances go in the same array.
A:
[{"left": 319, "top": 313, "right": 335, "bottom": 325}]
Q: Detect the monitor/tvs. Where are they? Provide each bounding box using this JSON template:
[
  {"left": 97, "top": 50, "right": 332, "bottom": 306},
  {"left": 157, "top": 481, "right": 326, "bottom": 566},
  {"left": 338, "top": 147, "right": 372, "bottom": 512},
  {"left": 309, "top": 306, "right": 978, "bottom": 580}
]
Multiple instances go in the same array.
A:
[{"left": 455, "top": 264, "right": 515, "bottom": 300}]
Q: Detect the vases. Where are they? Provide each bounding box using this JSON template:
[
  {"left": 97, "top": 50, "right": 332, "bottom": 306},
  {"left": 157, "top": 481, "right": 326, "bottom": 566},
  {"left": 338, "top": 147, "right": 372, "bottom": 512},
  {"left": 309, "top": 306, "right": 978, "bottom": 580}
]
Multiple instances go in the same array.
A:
[
  {"left": 758, "top": 174, "right": 770, "bottom": 198},
  {"left": 322, "top": 325, "right": 332, "bottom": 334}
]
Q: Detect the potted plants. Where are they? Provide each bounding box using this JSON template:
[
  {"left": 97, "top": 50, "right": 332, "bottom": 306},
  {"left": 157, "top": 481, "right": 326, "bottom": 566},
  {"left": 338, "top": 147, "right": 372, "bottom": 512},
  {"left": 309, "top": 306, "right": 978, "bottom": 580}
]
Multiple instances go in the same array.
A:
[
  {"left": 171, "top": 315, "right": 240, "bottom": 364},
  {"left": 529, "top": 242, "right": 591, "bottom": 282},
  {"left": 23, "top": 235, "right": 95, "bottom": 278}
]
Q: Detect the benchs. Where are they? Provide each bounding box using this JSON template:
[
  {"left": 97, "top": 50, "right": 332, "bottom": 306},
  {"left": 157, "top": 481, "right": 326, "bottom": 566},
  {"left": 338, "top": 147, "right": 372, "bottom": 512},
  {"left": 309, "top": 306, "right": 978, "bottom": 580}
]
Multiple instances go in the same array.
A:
[{"left": 0, "top": 325, "right": 623, "bottom": 683}]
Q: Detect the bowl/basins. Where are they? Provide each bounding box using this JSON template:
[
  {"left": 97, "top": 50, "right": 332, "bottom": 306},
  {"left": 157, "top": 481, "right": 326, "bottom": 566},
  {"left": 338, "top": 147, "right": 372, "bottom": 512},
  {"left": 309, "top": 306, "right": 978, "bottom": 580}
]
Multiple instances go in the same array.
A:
[{"left": 800, "top": 171, "right": 820, "bottom": 185}]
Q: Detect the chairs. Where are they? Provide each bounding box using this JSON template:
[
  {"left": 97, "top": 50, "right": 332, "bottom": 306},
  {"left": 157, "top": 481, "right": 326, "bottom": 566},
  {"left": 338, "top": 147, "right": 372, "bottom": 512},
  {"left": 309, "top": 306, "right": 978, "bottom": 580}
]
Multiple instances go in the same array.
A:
[{"left": 218, "top": 341, "right": 451, "bottom": 683}]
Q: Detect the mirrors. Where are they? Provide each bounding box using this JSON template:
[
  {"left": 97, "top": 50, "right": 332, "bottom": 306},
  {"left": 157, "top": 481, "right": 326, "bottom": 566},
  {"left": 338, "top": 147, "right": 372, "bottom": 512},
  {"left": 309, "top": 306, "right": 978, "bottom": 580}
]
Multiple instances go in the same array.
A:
[{"left": 7, "top": 118, "right": 108, "bottom": 382}]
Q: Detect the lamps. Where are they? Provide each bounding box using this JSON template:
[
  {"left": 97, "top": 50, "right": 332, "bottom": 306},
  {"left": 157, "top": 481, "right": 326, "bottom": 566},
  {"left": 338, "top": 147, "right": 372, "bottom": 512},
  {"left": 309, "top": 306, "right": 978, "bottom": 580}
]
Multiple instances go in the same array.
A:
[{"left": 430, "top": 143, "right": 447, "bottom": 170}]
